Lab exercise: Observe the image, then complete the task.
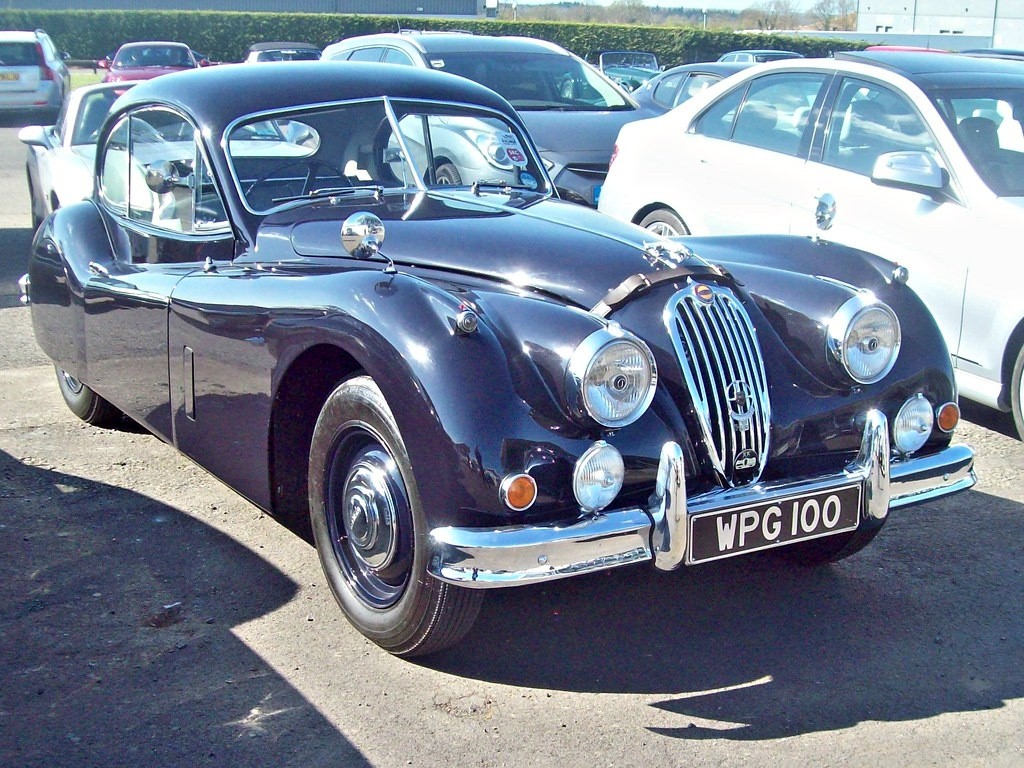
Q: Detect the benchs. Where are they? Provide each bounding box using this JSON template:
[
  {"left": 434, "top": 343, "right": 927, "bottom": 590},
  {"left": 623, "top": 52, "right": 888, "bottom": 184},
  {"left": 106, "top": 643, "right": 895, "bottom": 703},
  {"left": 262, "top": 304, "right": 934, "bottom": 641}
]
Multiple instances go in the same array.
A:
[{"left": 158, "top": 155, "right": 348, "bottom": 230}]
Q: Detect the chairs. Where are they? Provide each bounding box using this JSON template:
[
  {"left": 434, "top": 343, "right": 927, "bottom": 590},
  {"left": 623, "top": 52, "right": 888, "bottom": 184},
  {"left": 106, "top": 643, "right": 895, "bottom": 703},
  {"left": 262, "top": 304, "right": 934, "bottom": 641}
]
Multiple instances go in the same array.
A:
[
  {"left": 840, "top": 98, "right": 922, "bottom": 170},
  {"left": 953, "top": 117, "right": 1018, "bottom": 192},
  {"left": 734, "top": 102, "right": 801, "bottom": 157}
]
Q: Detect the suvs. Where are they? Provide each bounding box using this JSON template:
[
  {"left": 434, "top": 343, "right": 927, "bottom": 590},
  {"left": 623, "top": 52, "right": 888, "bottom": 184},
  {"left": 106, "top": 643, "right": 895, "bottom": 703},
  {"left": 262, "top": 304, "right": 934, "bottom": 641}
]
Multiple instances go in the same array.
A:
[{"left": 0, "top": 29, "right": 73, "bottom": 122}]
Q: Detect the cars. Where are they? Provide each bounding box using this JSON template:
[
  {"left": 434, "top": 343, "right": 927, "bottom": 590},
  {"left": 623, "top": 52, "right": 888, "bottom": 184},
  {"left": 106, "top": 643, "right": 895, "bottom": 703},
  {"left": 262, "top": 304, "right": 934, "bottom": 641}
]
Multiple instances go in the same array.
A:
[
  {"left": 97, "top": 40, "right": 218, "bottom": 110},
  {"left": 315, "top": 30, "right": 665, "bottom": 223},
  {"left": 16, "top": 58, "right": 982, "bottom": 656},
  {"left": 242, "top": 42, "right": 320, "bottom": 134},
  {"left": 588, "top": 43, "right": 1024, "bottom": 162},
  {"left": 598, "top": 51, "right": 1024, "bottom": 437}
]
